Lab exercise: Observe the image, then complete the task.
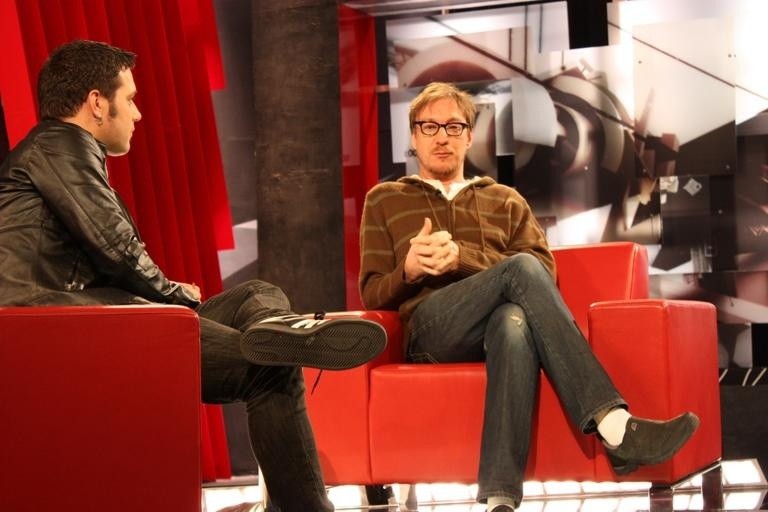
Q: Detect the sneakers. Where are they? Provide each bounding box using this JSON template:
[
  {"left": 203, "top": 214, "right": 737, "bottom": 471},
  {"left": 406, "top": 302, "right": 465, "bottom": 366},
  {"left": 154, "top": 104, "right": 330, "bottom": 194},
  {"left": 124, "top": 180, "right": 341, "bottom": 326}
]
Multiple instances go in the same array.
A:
[
  {"left": 239, "top": 314, "right": 387, "bottom": 370},
  {"left": 601, "top": 412, "right": 700, "bottom": 475}
]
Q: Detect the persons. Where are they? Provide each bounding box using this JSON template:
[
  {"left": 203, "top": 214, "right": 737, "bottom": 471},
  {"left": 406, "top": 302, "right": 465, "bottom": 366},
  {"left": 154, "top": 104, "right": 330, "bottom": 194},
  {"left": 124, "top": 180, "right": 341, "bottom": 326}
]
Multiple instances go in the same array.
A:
[
  {"left": 1, "top": 42, "right": 388, "bottom": 511},
  {"left": 357, "top": 82, "right": 700, "bottom": 512}
]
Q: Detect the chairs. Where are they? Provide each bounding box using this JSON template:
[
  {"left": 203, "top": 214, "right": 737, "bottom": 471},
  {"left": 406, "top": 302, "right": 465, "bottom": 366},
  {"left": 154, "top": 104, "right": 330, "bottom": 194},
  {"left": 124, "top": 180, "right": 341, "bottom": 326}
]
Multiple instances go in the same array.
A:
[
  {"left": 300, "top": 241, "right": 723, "bottom": 510},
  {"left": 0, "top": 303, "right": 231, "bottom": 512}
]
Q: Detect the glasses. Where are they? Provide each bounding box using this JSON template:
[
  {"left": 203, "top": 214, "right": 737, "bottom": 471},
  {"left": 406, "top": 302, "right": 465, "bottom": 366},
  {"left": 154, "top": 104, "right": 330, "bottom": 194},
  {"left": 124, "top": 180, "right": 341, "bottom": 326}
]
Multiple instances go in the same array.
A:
[{"left": 415, "top": 121, "right": 469, "bottom": 136}]
